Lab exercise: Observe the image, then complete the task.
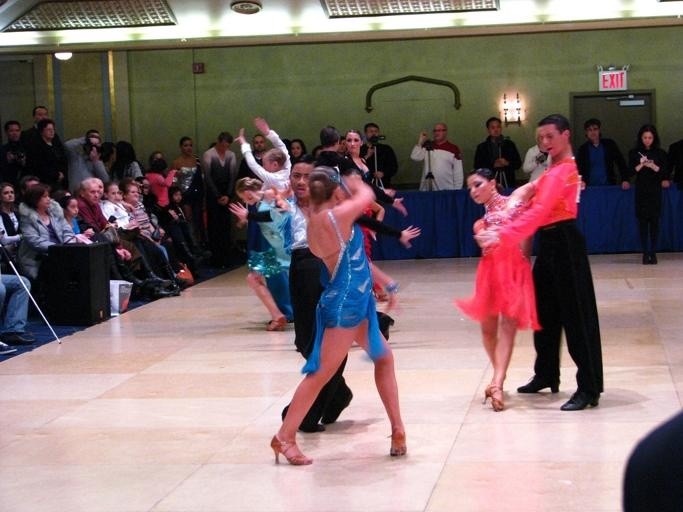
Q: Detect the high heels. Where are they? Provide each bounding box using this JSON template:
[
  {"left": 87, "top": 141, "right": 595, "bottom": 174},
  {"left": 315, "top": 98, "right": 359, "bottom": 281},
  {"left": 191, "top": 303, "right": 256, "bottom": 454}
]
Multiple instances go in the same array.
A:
[
  {"left": 482, "top": 385, "right": 504, "bottom": 412},
  {"left": 382, "top": 315, "right": 393, "bottom": 341},
  {"left": 560, "top": 392, "right": 600, "bottom": 411},
  {"left": 266, "top": 316, "right": 287, "bottom": 331},
  {"left": 272, "top": 436, "right": 313, "bottom": 465},
  {"left": 517, "top": 376, "right": 559, "bottom": 393},
  {"left": 391, "top": 427, "right": 407, "bottom": 456}
]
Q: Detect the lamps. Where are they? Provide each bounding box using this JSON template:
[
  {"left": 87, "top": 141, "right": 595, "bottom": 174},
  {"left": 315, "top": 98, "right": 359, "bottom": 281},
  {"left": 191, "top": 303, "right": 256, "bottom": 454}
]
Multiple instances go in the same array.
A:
[{"left": 498, "top": 91, "right": 525, "bottom": 127}]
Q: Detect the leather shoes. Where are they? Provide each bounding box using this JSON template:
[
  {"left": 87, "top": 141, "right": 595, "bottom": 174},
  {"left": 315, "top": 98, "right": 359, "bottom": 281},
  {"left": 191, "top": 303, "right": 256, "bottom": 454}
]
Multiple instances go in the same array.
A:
[
  {"left": 133, "top": 271, "right": 181, "bottom": 298},
  {"left": 2, "top": 335, "right": 34, "bottom": 345},
  {"left": 320, "top": 393, "right": 354, "bottom": 425},
  {"left": 283, "top": 417, "right": 323, "bottom": 432}
]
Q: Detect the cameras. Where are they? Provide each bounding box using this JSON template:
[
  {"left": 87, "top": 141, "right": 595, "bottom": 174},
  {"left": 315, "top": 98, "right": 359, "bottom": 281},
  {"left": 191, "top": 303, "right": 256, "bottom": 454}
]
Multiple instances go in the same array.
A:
[
  {"left": 423, "top": 140, "right": 432, "bottom": 150},
  {"left": 369, "top": 135, "right": 385, "bottom": 146},
  {"left": 11, "top": 151, "right": 24, "bottom": 159},
  {"left": 536, "top": 152, "right": 547, "bottom": 163},
  {"left": 84, "top": 143, "right": 102, "bottom": 153},
  {"left": 495, "top": 134, "right": 505, "bottom": 144}
]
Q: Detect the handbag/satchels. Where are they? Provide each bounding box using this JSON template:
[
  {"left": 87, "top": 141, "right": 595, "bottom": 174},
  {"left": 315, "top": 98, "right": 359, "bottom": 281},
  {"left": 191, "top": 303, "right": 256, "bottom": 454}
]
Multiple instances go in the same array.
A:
[{"left": 109, "top": 279, "right": 133, "bottom": 316}]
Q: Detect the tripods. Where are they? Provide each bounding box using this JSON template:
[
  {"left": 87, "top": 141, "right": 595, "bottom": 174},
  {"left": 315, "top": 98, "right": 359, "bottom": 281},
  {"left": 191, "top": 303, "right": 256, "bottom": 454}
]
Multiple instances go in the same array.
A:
[
  {"left": 420, "top": 150, "right": 440, "bottom": 191},
  {"left": 494, "top": 145, "right": 508, "bottom": 188},
  {"left": 370, "top": 146, "right": 385, "bottom": 190}
]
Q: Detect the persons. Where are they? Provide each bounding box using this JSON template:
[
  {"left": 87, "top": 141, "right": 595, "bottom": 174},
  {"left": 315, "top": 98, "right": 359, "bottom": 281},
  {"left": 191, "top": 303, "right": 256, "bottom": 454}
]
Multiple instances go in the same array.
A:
[
  {"left": 0, "top": 99, "right": 200, "bottom": 355},
  {"left": 470, "top": 112, "right": 605, "bottom": 411},
  {"left": 205, "top": 116, "right": 421, "bottom": 342},
  {"left": 625, "top": 121, "right": 676, "bottom": 265},
  {"left": 412, "top": 111, "right": 682, "bottom": 207},
  {"left": 271, "top": 157, "right": 408, "bottom": 466},
  {"left": 452, "top": 164, "right": 587, "bottom": 413}
]
